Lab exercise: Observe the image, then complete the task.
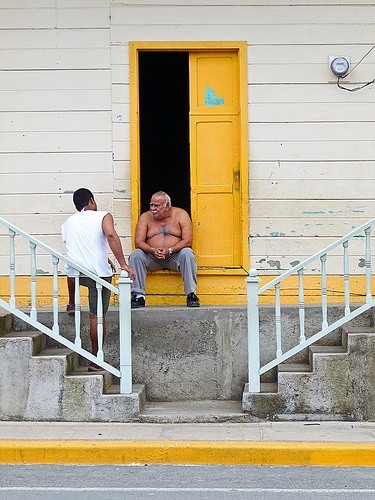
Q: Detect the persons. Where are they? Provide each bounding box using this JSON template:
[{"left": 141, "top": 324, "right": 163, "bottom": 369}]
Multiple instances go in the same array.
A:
[
  {"left": 62, "top": 188, "right": 136, "bottom": 372},
  {"left": 127, "top": 191, "right": 201, "bottom": 309}
]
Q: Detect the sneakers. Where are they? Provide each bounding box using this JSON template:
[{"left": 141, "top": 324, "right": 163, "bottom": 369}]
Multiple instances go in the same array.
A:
[
  {"left": 131, "top": 294, "right": 146, "bottom": 308},
  {"left": 186, "top": 293, "right": 200, "bottom": 307},
  {"left": 67, "top": 304, "right": 75, "bottom": 314},
  {"left": 88, "top": 360, "right": 110, "bottom": 371}
]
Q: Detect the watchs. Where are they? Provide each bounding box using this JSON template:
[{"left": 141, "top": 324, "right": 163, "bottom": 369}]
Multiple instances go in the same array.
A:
[{"left": 168, "top": 248, "right": 173, "bottom": 257}]
